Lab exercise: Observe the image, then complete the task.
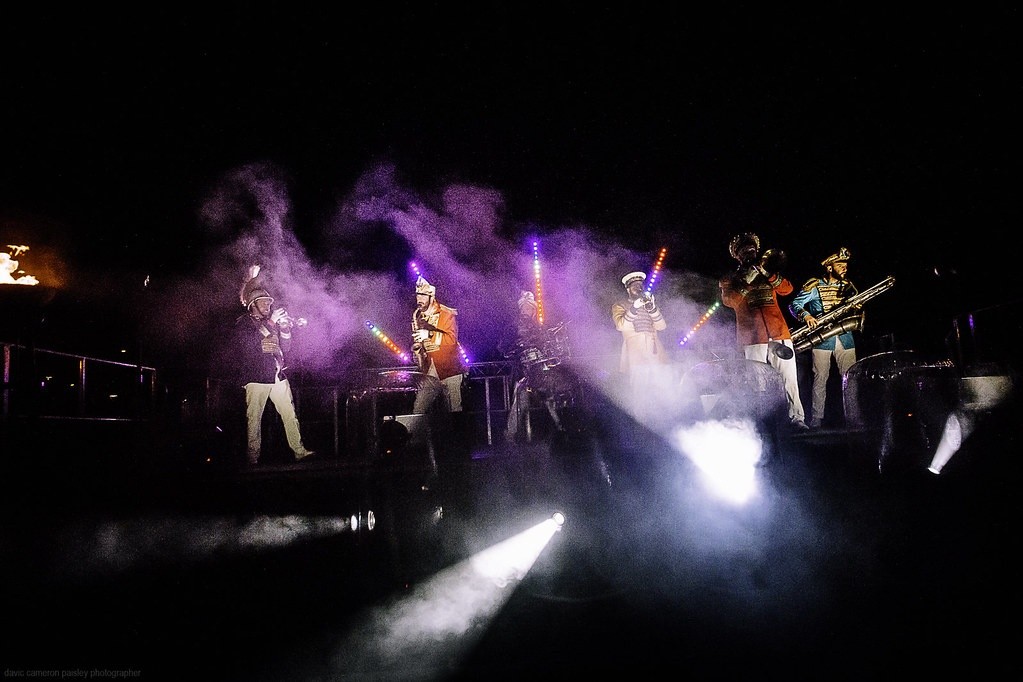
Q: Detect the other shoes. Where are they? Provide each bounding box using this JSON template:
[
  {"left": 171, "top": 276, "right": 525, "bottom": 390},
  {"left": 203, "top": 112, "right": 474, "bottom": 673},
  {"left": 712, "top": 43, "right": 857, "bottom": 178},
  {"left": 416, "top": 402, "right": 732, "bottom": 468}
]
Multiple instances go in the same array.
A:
[
  {"left": 846, "top": 417, "right": 868, "bottom": 432},
  {"left": 809, "top": 418, "right": 821, "bottom": 431},
  {"left": 789, "top": 420, "right": 810, "bottom": 434},
  {"left": 249, "top": 453, "right": 260, "bottom": 464},
  {"left": 294, "top": 447, "right": 317, "bottom": 461}
]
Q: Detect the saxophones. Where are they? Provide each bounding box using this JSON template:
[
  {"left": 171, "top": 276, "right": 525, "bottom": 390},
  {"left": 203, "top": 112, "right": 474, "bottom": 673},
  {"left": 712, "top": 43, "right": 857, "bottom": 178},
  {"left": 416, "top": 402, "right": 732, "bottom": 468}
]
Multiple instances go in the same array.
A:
[
  {"left": 787, "top": 275, "right": 896, "bottom": 356},
  {"left": 410, "top": 303, "right": 428, "bottom": 373}
]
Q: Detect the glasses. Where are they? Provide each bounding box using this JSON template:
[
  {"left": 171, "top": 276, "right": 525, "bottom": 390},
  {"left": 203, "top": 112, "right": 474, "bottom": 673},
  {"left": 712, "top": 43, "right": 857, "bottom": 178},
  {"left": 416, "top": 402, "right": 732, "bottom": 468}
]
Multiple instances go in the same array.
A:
[{"left": 628, "top": 283, "right": 646, "bottom": 290}]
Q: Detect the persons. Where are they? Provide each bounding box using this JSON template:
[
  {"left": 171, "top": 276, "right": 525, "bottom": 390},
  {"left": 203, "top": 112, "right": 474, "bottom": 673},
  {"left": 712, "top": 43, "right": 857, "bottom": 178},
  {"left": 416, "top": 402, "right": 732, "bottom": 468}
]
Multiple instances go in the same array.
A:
[
  {"left": 412, "top": 284, "right": 463, "bottom": 415},
  {"left": 496, "top": 299, "right": 566, "bottom": 440},
  {"left": 789, "top": 252, "right": 866, "bottom": 428},
  {"left": 721, "top": 232, "right": 809, "bottom": 431},
  {"left": 234, "top": 288, "right": 315, "bottom": 463},
  {"left": 612, "top": 272, "right": 667, "bottom": 396}
]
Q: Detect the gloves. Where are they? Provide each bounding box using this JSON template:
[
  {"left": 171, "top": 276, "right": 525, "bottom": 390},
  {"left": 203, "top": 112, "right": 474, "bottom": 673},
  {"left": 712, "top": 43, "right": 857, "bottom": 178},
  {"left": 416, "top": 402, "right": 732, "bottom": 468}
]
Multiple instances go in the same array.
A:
[
  {"left": 644, "top": 291, "right": 656, "bottom": 312},
  {"left": 413, "top": 352, "right": 425, "bottom": 366},
  {"left": 634, "top": 297, "right": 650, "bottom": 309},
  {"left": 270, "top": 308, "right": 285, "bottom": 323},
  {"left": 412, "top": 329, "right": 429, "bottom": 343},
  {"left": 276, "top": 311, "right": 288, "bottom": 327}
]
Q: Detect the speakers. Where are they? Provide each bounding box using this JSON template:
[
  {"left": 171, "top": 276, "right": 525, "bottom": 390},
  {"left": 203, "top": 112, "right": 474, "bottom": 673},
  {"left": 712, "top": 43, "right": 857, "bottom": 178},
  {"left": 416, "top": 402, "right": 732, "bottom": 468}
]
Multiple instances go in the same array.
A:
[{"left": 381, "top": 414, "right": 429, "bottom": 456}]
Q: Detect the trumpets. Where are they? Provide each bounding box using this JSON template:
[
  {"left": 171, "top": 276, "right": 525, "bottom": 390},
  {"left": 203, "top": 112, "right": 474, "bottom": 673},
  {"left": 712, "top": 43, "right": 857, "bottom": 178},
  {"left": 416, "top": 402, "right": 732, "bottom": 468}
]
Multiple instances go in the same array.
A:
[
  {"left": 269, "top": 307, "right": 308, "bottom": 331},
  {"left": 639, "top": 289, "right": 653, "bottom": 311}
]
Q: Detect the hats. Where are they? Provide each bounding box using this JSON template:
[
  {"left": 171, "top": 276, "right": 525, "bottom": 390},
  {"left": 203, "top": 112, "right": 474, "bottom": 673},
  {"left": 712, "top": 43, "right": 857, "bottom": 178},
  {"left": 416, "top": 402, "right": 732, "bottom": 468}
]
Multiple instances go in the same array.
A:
[
  {"left": 621, "top": 272, "right": 646, "bottom": 289},
  {"left": 415, "top": 276, "right": 436, "bottom": 297},
  {"left": 240, "top": 266, "right": 274, "bottom": 311},
  {"left": 819, "top": 247, "right": 852, "bottom": 267},
  {"left": 728, "top": 231, "right": 760, "bottom": 259},
  {"left": 518, "top": 291, "right": 538, "bottom": 310}
]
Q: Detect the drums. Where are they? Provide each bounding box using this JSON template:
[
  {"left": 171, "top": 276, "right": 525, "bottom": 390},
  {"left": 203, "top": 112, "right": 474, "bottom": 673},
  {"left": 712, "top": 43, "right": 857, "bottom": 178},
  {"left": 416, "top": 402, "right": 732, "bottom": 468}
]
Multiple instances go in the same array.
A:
[{"left": 522, "top": 340, "right": 560, "bottom": 366}]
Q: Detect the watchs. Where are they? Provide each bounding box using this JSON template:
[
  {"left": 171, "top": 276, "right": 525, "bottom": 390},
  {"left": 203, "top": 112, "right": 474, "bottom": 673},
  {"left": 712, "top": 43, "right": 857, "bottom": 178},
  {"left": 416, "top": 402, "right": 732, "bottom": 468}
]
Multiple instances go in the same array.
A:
[{"left": 428, "top": 331, "right": 433, "bottom": 338}]
